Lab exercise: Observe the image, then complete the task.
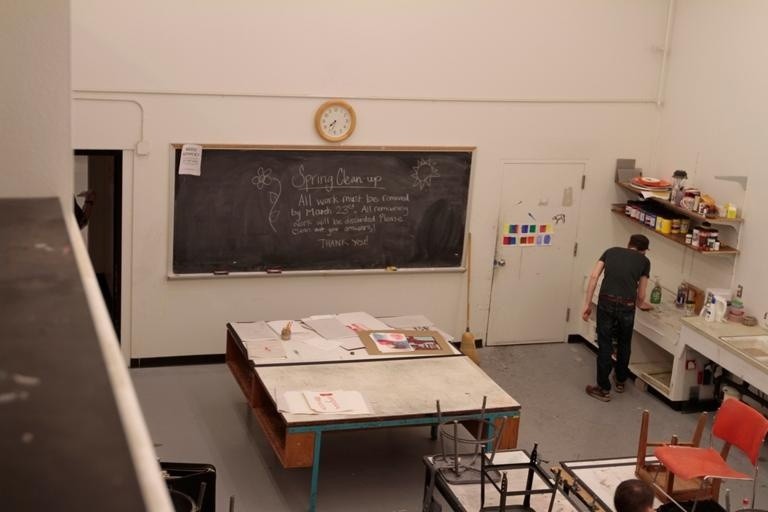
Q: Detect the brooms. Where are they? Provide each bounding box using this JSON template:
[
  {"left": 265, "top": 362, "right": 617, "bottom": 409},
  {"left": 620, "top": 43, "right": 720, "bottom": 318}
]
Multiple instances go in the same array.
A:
[{"left": 460, "top": 232, "right": 482, "bottom": 368}]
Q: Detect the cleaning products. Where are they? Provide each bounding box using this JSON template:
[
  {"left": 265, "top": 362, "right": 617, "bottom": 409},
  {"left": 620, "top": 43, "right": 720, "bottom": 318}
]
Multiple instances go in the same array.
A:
[{"left": 704, "top": 297, "right": 716, "bottom": 323}]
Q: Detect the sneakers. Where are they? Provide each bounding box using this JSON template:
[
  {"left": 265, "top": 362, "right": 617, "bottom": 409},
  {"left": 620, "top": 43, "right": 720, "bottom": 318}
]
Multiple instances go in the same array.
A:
[
  {"left": 613, "top": 374, "right": 624, "bottom": 392},
  {"left": 586, "top": 385, "right": 610, "bottom": 402}
]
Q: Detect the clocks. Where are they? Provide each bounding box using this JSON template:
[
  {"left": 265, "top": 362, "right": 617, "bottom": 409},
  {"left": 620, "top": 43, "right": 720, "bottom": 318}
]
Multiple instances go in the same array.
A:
[{"left": 314, "top": 100, "right": 355, "bottom": 141}]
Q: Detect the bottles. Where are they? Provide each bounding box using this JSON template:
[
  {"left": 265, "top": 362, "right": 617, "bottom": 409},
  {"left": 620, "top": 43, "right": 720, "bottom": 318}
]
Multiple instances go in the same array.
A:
[
  {"left": 677, "top": 286, "right": 715, "bottom": 322},
  {"left": 281, "top": 328, "right": 291, "bottom": 340},
  {"left": 624, "top": 169, "right": 737, "bottom": 253},
  {"left": 650, "top": 282, "right": 663, "bottom": 306},
  {"left": 736, "top": 498, "right": 750, "bottom": 510}
]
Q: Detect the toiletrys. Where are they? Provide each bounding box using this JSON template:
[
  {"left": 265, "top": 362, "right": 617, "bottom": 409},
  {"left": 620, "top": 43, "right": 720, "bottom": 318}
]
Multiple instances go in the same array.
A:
[
  {"left": 651, "top": 277, "right": 662, "bottom": 303},
  {"left": 729, "top": 284, "right": 745, "bottom": 315}
]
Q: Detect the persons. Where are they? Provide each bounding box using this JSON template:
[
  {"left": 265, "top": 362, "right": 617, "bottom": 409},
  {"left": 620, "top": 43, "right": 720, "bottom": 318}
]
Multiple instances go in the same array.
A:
[
  {"left": 581, "top": 234, "right": 653, "bottom": 402},
  {"left": 613, "top": 479, "right": 654, "bottom": 512}
]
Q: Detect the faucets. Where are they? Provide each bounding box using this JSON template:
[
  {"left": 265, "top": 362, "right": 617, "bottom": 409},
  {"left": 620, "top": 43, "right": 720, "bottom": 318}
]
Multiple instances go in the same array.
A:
[{"left": 763, "top": 312, "right": 767, "bottom": 319}]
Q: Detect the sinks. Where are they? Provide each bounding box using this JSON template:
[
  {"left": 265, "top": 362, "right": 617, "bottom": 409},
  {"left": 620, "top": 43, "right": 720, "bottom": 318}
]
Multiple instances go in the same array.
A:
[{"left": 720, "top": 332, "right": 768, "bottom": 376}]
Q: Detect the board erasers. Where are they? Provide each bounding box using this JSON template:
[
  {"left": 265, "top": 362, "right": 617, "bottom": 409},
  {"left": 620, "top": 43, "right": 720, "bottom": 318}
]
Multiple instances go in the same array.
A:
[
  {"left": 268, "top": 270, "right": 281, "bottom": 273},
  {"left": 214, "top": 271, "right": 228, "bottom": 274}
]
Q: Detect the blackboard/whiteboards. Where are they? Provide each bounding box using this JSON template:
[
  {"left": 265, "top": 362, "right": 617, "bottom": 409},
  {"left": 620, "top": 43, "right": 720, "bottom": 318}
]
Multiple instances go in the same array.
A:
[{"left": 168, "top": 143, "right": 477, "bottom": 280}]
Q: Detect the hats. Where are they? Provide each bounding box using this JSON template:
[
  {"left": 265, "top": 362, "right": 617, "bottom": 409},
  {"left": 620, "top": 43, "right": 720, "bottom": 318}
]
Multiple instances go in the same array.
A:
[{"left": 630, "top": 235, "right": 648, "bottom": 251}]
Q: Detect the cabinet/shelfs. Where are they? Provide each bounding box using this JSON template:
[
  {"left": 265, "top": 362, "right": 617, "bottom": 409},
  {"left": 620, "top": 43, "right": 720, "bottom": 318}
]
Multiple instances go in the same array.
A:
[
  {"left": 584, "top": 275, "right": 731, "bottom": 401},
  {"left": 612, "top": 182, "right": 741, "bottom": 254}
]
